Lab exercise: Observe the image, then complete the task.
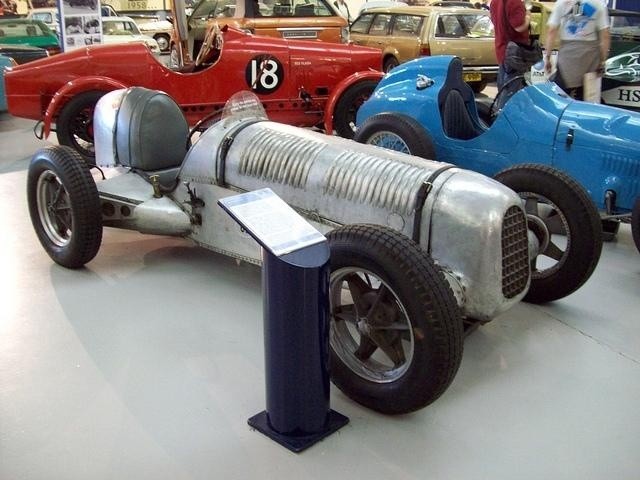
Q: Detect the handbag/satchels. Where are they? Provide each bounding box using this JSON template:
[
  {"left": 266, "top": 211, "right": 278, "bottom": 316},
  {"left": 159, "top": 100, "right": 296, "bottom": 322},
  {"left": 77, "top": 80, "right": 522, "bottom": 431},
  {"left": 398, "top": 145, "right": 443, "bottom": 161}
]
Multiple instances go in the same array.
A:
[{"left": 502, "top": 40, "right": 543, "bottom": 74}]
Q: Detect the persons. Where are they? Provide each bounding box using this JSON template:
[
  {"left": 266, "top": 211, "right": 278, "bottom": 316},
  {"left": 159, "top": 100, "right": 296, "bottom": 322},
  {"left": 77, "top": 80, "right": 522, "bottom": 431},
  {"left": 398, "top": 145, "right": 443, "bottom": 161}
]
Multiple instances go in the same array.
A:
[
  {"left": 334, "top": 0, "right": 351, "bottom": 44},
  {"left": 490, "top": 0, "right": 544, "bottom": 109},
  {"left": 544, "top": 0, "right": 611, "bottom": 103},
  {"left": 474, "top": 2, "right": 489, "bottom": 19}
]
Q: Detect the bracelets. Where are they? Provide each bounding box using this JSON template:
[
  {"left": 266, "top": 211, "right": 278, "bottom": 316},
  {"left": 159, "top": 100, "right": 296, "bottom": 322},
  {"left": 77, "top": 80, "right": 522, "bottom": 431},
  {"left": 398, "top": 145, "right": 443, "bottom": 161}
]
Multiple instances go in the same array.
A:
[{"left": 545, "top": 56, "right": 551, "bottom": 61}]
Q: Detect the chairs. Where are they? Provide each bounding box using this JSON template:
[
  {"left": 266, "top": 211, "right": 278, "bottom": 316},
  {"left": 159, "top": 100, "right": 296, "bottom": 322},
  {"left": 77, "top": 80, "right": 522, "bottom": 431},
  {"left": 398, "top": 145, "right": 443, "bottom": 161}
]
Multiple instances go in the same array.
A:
[
  {"left": 444, "top": 89, "right": 479, "bottom": 138},
  {"left": 115, "top": 86, "right": 194, "bottom": 189}
]
